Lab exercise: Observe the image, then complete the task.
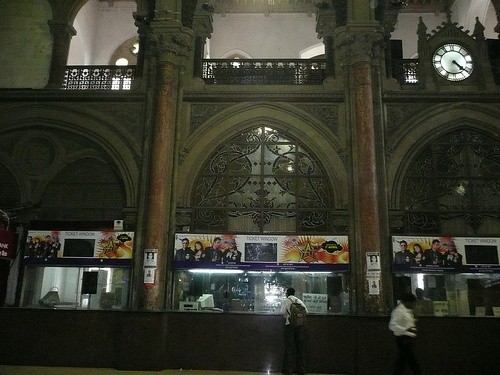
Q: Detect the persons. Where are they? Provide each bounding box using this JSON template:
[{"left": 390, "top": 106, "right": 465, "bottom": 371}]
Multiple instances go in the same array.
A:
[
  {"left": 415, "top": 288, "right": 432, "bottom": 303},
  {"left": 394, "top": 239, "right": 463, "bottom": 268},
  {"left": 388, "top": 291, "right": 423, "bottom": 375},
  {"left": 280, "top": 287, "right": 309, "bottom": 375},
  {"left": 174, "top": 237, "right": 242, "bottom": 264},
  {"left": 23, "top": 235, "right": 62, "bottom": 261}
]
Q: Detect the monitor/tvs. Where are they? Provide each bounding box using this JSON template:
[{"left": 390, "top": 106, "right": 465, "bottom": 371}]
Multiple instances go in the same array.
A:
[{"left": 198, "top": 294, "right": 214, "bottom": 308}]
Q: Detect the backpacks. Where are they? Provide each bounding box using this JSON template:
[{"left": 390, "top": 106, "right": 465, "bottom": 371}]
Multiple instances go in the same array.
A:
[{"left": 286, "top": 298, "right": 306, "bottom": 329}]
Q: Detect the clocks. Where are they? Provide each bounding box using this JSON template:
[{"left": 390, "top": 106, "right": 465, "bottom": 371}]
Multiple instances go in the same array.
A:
[{"left": 430, "top": 42, "right": 475, "bottom": 82}]
